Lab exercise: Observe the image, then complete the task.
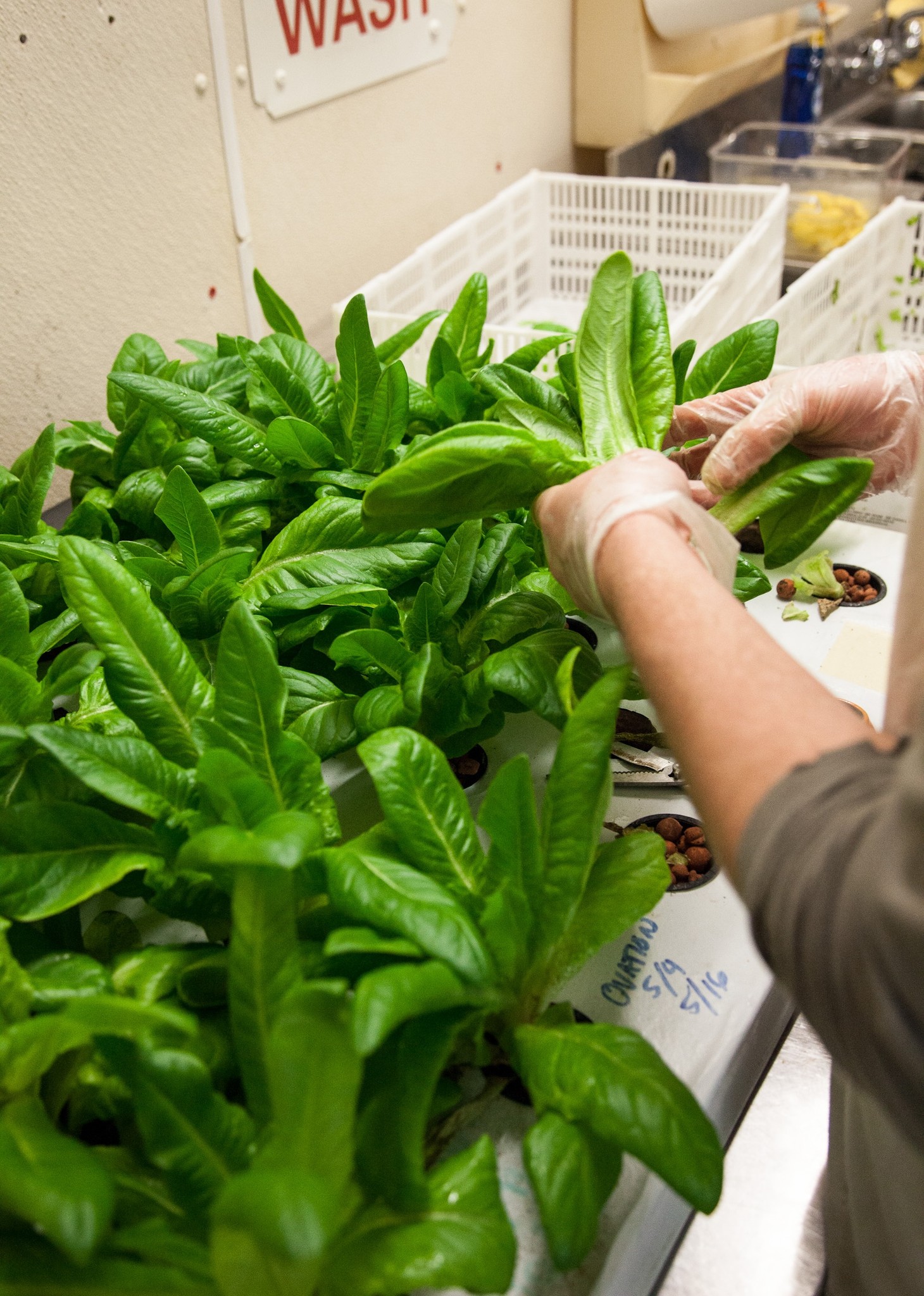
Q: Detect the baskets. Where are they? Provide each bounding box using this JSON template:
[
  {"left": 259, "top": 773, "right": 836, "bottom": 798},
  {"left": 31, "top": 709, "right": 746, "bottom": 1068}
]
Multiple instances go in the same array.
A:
[
  {"left": 752, "top": 195, "right": 924, "bottom": 373},
  {"left": 331, "top": 167, "right": 789, "bottom": 381}
]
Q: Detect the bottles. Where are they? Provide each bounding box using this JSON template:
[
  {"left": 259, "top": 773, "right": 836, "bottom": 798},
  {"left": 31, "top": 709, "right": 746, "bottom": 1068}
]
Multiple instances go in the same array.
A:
[{"left": 773, "top": 28, "right": 827, "bottom": 177}]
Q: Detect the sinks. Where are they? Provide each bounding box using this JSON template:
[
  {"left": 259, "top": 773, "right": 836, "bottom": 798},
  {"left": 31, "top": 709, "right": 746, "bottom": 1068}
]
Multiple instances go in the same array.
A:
[
  {"left": 813, "top": 136, "right": 924, "bottom": 184},
  {"left": 833, "top": 89, "right": 924, "bottom": 133}
]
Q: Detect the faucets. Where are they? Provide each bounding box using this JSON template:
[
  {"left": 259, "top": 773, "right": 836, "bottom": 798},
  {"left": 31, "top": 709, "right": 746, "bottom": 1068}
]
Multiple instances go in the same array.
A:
[{"left": 824, "top": 16, "right": 921, "bottom": 82}]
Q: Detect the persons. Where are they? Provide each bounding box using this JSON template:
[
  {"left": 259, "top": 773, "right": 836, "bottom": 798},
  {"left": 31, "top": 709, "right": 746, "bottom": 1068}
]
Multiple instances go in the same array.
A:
[{"left": 533, "top": 343, "right": 924, "bottom": 1296}]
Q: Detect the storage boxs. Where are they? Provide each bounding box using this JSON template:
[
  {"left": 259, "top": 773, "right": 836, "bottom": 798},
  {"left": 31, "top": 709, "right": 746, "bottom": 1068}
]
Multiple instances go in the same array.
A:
[{"left": 335, "top": 121, "right": 923, "bottom": 404}]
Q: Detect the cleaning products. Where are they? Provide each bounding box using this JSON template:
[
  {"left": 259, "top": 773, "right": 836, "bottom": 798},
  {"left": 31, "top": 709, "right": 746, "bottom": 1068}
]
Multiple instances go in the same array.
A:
[{"left": 776, "top": 43, "right": 825, "bottom": 171}]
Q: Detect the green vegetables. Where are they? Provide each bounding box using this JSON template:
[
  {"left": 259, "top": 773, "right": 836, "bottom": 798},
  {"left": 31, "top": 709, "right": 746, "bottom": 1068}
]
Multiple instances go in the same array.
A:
[{"left": 0, "top": 254, "right": 876, "bottom": 1296}]
